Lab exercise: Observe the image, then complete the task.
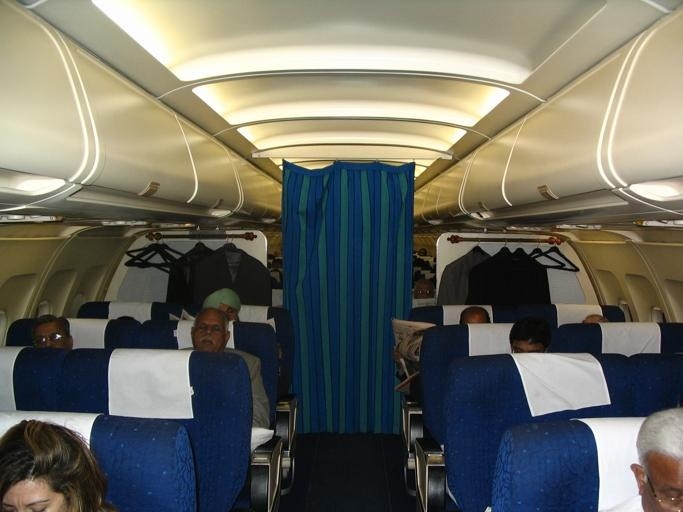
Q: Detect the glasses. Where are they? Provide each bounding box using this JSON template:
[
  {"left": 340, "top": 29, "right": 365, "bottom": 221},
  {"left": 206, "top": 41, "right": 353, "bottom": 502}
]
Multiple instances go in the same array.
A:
[
  {"left": 32, "top": 332, "right": 65, "bottom": 348},
  {"left": 412, "top": 290, "right": 433, "bottom": 297},
  {"left": 644, "top": 473, "right": 683, "bottom": 512}
]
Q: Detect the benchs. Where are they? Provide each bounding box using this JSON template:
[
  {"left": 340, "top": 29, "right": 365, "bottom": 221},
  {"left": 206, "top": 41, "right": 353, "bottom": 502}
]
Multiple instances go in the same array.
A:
[
  {"left": 400, "top": 304, "right": 680, "bottom": 511},
  {"left": 0, "top": 301, "right": 294, "bottom": 512}
]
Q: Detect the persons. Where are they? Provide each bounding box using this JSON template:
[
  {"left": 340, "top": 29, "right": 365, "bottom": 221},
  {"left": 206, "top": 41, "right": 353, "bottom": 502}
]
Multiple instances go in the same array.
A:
[
  {"left": 173, "top": 307, "right": 270, "bottom": 430},
  {"left": 0, "top": 419, "right": 104, "bottom": 512},
  {"left": 414, "top": 281, "right": 430, "bottom": 299},
  {"left": 581, "top": 314, "right": 609, "bottom": 323},
  {"left": 509, "top": 318, "right": 552, "bottom": 353},
  {"left": 459, "top": 306, "right": 492, "bottom": 325},
  {"left": 203, "top": 288, "right": 245, "bottom": 321},
  {"left": 30, "top": 315, "right": 73, "bottom": 352},
  {"left": 602, "top": 409, "right": 683, "bottom": 512}
]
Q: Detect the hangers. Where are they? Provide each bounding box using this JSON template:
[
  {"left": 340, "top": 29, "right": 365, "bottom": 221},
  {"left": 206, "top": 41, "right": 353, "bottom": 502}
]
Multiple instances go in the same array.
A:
[
  {"left": 124, "top": 233, "right": 262, "bottom": 273},
  {"left": 442, "top": 238, "right": 580, "bottom": 273}
]
